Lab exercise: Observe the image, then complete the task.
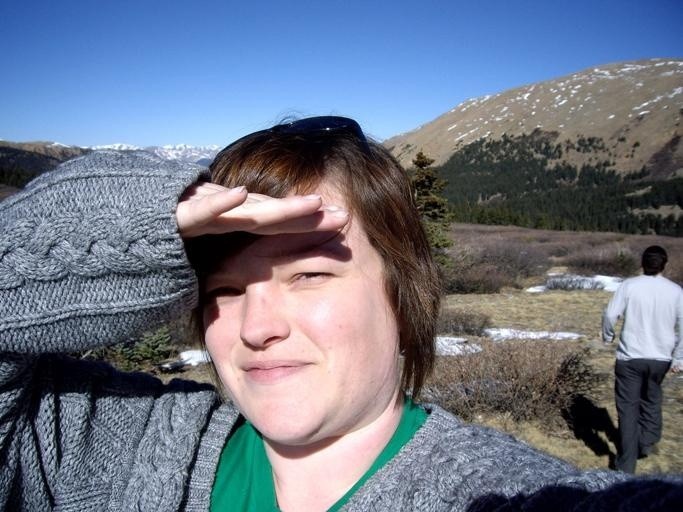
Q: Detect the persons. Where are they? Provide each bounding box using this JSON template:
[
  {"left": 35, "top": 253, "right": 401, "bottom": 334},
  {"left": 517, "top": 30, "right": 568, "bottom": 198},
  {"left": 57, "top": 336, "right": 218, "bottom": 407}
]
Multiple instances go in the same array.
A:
[
  {"left": 0, "top": 115, "right": 683, "bottom": 512},
  {"left": 601, "top": 245, "right": 683, "bottom": 474}
]
